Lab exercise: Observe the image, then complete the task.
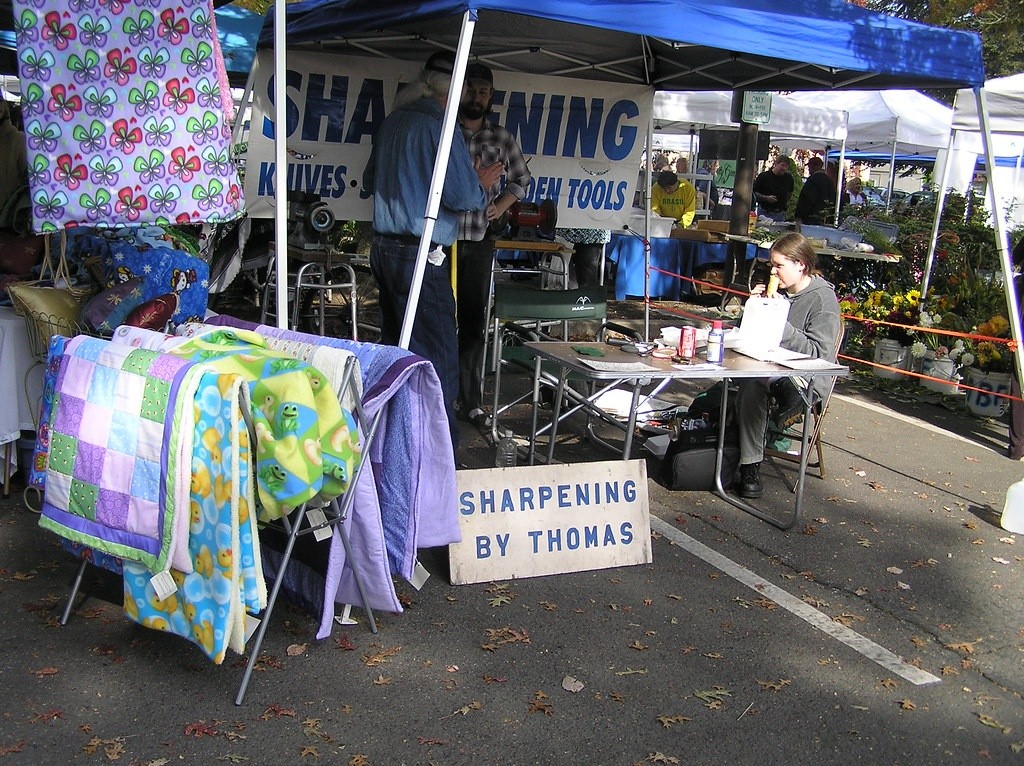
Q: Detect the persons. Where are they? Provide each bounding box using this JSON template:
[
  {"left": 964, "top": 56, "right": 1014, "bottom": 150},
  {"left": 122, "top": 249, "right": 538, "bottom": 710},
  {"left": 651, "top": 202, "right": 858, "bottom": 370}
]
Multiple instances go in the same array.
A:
[
  {"left": 695, "top": 159, "right": 719, "bottom": 220},
  {"left": 456, "top": 62, "right": 531, "bottom": 430},
  {"left": 362, "top": 51, "right": 504, "bottom": 473},
  {"left": 839, "top": 177, "right": 866, "bottom": 213},
  {"left": 651, "top": 171, "right": 696, "bottom": 229},
  {"left": 677, "top": 158, "right": 688, "bottom": 173},
  {"left": 753, "top": 155, "right": 794, "bottom": 222},
  {"left": 728, "top": 233, "right": 841, "bottom": 497},
  {"left": 794, "top": 158, "right": 836, "bottom": 225}
]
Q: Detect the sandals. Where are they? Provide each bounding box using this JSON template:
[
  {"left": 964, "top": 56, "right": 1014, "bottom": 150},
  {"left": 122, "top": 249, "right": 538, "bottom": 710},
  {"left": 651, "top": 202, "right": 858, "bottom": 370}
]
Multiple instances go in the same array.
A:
[{"left": 455, "top": 405, "right": 498, "bottom": 429}]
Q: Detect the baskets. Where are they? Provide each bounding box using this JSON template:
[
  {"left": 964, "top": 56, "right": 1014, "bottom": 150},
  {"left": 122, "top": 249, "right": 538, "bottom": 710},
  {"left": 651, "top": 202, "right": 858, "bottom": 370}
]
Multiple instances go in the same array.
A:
[{"left": 6, "top": 225, "right": 109, "bottom": 317}]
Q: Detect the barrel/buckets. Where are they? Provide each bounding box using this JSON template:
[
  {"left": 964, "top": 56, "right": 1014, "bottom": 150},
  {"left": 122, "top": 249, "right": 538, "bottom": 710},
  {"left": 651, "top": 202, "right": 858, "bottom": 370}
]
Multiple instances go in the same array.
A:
[
  {"left": 872, "top": 337, "right": 912, "bottom": 382},
  {"left": 919, "top": 348, "right": 960, "bottom": 393},
  {"left": 965, "top": 367, "right": 1011, "bottom": 415}
]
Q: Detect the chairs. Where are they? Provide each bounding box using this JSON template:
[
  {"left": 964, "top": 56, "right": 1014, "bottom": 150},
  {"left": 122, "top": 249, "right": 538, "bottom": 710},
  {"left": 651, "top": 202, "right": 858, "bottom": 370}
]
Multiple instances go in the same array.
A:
[
  {"left": 725, "top": 321, "right": 847, "bottom": 493},
  {"left": 493, "top": 281, "right": 644, "bottom": 461}
]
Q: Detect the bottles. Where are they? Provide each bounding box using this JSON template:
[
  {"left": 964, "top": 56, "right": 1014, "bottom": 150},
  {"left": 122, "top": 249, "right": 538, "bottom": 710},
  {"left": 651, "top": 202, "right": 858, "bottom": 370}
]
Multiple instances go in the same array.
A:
[
  {"left": 1001, "top": 479, "right": 1024, "bottom": 535},
  {"left": 496, "top": 430, "right": 517, "bottom": 468},
  {"left": 706, "top": 321, "right": 724, "bottom": 363}
]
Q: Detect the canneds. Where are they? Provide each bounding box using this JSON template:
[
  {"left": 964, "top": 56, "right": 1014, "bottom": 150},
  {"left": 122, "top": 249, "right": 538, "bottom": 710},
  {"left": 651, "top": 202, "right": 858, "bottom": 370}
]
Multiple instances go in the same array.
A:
[{"left": 679, "top": 326, "right": 696, "bottom": 358}]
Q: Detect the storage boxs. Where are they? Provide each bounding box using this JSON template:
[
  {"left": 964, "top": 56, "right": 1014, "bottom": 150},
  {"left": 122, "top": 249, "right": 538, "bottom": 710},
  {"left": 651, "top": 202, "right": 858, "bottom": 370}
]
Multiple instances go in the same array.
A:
[
  {"left": 612, "top": 215, "right": 676, "bottom": 238},
  {"left": 671, "top": 219, "right": 730, "bottom": 243}
]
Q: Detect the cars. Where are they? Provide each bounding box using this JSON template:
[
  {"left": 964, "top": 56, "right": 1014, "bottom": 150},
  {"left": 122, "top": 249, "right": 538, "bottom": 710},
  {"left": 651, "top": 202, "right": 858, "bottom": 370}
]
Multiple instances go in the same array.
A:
[{"left": 845, "top": 187, "right": 939, "bottom": 216}]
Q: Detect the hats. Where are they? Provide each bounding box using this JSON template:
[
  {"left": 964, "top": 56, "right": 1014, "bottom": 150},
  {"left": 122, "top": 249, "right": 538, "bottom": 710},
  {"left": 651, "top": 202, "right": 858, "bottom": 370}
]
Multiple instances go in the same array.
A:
[
  {"left": 465, "top": 63, "right": 494, "bottom": 88},
  {"left": 804, "top": 157, "right": 823, "bottom": 167},
  {"left": 425, "top": 51, "right": 455, "bottom": 75}
]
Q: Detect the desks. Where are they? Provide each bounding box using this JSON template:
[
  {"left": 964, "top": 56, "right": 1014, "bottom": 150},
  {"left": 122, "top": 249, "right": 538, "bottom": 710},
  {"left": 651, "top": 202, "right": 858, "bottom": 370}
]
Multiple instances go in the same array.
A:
[
  {"left": 556, "top": 227, "right": 611, "bottom": 286},
  {"left": 0, "top": 306, "right": 49, "bottom": 499},
  {"left": 521, "top": 339, "right": 851, "bottom": 530},
  {"left": 729, "top": 235, "right": 899, "bottom": 288},
  {"left": 605, "top": 233, "right": 757, "bottom": 303}
]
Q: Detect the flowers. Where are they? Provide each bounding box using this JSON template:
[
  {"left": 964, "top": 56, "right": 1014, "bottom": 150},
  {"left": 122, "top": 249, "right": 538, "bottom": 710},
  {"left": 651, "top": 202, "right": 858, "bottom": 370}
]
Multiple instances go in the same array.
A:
[{"left": 835, "top": 253, "right": 1012, "bottom": 377}]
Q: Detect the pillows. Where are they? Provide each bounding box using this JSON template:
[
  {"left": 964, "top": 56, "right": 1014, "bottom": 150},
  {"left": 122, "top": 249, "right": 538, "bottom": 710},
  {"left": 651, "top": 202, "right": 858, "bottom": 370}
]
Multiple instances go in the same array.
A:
[{"left": 5, "top": 273, "right": 181, "bottom": 351}]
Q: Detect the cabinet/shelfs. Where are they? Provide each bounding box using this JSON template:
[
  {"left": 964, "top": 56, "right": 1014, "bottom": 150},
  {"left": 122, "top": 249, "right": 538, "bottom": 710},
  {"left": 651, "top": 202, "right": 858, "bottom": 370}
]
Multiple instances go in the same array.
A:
[{"left": 639, "top": 171, "right": 713, "bottom": 220}]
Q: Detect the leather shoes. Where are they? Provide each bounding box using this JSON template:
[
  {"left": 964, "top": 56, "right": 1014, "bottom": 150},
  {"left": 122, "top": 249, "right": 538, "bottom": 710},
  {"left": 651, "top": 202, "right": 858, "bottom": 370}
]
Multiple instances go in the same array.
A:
[{"left": 740, "top": 462, "right": 763, "bottom": 497}]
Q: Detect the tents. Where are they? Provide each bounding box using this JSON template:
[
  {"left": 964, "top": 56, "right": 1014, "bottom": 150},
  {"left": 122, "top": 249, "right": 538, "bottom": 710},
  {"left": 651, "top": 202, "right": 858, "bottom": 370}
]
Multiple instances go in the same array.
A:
[{"left": 196, "top": 0, "right": 1024, "bottom": 534}]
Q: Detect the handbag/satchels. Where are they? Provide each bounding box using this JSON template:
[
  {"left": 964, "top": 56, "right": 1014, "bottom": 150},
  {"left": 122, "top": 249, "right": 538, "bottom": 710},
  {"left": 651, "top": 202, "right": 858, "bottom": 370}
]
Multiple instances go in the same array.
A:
[{"left": 663, "top": 425, "right": 740, "bottom": 491}]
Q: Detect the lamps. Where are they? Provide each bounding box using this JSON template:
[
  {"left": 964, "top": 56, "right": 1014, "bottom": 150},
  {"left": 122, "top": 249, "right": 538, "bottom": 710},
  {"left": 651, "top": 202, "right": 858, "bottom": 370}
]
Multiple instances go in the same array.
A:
[{"left": 654, "top": 120, "right": 661, "bottom": 130}]
